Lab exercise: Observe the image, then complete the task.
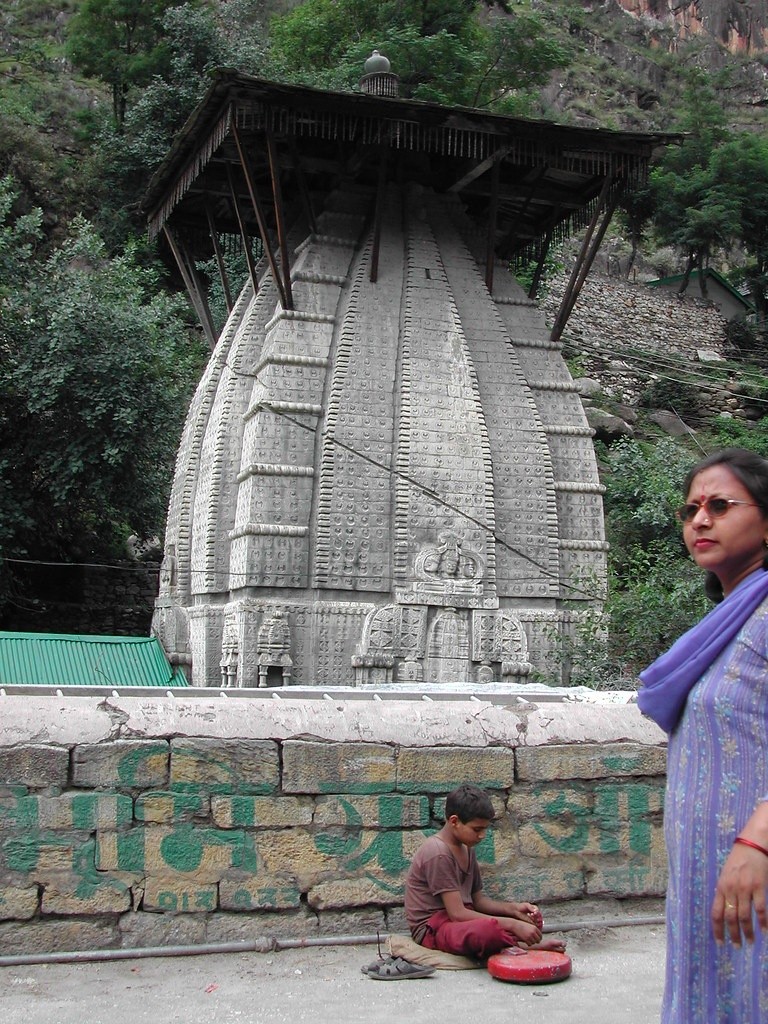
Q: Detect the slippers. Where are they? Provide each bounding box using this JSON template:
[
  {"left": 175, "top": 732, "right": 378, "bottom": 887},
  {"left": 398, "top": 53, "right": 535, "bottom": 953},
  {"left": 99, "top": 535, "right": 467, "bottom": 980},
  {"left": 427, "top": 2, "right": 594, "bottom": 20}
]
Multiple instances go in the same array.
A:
[
  {"left": 361, "top": 956, "right": 398, "bottom": 974},
  {"left": 369, "top": 958, "right": 437, "bottom": 980}
]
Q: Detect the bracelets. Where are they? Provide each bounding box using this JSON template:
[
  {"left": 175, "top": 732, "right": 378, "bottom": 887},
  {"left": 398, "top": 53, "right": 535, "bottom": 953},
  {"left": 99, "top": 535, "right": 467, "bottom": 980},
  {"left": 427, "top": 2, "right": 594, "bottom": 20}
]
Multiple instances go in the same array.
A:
[{"left": 732, "top": 836, "right": 768, "bottom": 859}]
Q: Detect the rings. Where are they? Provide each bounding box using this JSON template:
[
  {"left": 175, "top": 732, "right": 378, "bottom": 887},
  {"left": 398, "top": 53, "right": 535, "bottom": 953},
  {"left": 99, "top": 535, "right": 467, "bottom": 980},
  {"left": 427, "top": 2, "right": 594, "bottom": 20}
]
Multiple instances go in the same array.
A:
[{"left": 723, "top": 898, "right": 739, "bottom": 912}]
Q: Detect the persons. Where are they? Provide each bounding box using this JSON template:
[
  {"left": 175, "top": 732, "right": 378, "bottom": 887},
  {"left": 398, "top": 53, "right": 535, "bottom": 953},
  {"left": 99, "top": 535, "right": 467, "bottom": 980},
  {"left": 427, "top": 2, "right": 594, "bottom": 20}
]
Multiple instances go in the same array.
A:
[
  {"left": 407, "top": 786, "right": 567, "bottom": 959},
  {"left": 634, "top": 447, "right": 768, "bottom": 1024}
]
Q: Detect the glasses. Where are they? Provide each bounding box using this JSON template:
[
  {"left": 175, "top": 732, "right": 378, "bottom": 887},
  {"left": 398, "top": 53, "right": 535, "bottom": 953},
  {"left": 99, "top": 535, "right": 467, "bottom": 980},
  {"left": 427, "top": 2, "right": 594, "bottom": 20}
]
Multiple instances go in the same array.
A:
[{"left": 675, "top": 498, "right": 768, "bottom": 522}]
